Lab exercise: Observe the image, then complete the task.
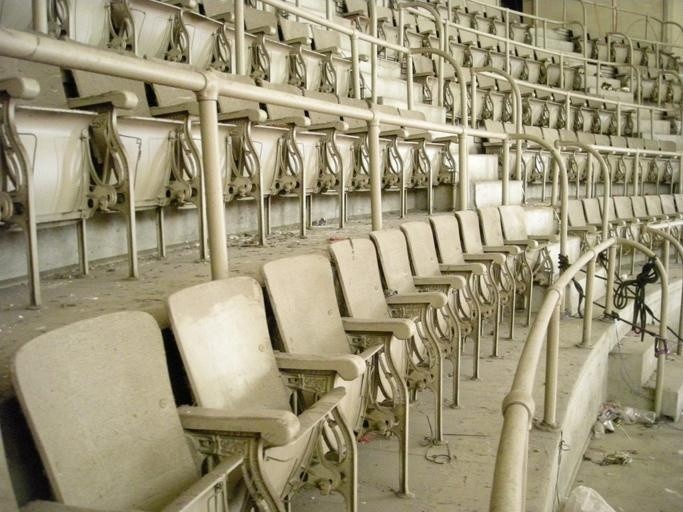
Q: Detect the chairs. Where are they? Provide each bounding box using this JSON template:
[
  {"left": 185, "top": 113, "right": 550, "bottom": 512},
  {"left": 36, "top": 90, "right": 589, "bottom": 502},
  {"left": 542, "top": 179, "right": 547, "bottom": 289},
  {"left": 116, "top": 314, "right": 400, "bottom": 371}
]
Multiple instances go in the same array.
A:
[
  {"left": 329, "top": 238, "right": 451, "bottom": 447},
  {"left": 10, "top": 309, "right": 300, "bottom": 511},
  {"left": 427, "top": 215, "right": 507, "bottom": 360},
  {"left": 477, "top": 206, "right": 539, "bottom": 327},
  {"left": 1, "top": 440, "right": 25, "bottom": 511},
  {"left": 399, "top": 222, "right": 486, "bottom": 383},
  {"left": 368, "top": 229, "right": 467, "bottom": 410},
  {"left": 168, "top": 277, "right": 366, "bottom": 512},
  {"left": 262, "top": 253, "right": 417, "bottom": 500},
  {"left": 456, "top": 211, "right": 521, "bottom": 341},
  {"left": 1, "top": 2, "right": 681, "bottom": 308}
]
